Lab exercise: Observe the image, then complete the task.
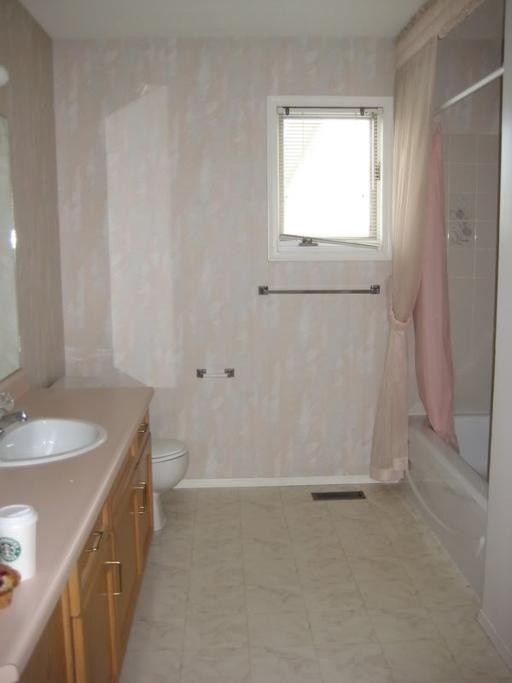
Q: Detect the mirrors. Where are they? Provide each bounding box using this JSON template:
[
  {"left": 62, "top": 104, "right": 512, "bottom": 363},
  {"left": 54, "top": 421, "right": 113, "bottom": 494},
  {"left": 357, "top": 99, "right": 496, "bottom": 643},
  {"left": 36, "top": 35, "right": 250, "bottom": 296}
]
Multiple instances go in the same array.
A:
[{"left": 1, "top": 116, "right": 21, "bottom": 385}]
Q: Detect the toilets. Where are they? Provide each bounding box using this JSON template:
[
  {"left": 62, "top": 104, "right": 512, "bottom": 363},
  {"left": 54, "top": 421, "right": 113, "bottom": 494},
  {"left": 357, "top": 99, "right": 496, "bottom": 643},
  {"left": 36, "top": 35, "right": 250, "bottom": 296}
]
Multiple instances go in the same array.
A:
[{"left": 50, "top": 375, "right": 188, "bottom": 531}]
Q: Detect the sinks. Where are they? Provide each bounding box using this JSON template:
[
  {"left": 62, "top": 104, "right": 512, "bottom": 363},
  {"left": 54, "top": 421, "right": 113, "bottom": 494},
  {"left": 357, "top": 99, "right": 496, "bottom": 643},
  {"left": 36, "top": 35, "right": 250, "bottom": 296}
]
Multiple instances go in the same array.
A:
[{"left": 0, "top": 416, "right": 108, "bottom": 466}]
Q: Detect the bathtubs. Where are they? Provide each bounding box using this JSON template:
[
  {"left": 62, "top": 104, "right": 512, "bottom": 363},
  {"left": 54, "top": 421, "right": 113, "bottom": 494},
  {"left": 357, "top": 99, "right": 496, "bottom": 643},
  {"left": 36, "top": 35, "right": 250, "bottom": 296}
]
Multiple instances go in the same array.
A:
[{"left": 403, "top": 413, "right": 491, "bottom": 606}]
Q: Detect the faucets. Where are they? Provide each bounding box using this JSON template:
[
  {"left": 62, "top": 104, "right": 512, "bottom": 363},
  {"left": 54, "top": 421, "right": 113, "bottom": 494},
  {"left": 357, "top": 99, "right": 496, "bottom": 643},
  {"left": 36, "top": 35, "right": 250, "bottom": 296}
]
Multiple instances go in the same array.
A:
[{"left": 0, "top": 391, "right": 27, "bottom": 432}]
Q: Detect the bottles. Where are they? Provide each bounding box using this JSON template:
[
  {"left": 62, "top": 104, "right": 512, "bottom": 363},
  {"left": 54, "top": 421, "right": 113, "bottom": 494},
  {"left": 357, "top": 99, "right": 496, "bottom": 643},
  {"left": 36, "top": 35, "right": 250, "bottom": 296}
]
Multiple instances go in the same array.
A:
[{"left": 0, "top": 504, "right": 38, "bottom": 583}]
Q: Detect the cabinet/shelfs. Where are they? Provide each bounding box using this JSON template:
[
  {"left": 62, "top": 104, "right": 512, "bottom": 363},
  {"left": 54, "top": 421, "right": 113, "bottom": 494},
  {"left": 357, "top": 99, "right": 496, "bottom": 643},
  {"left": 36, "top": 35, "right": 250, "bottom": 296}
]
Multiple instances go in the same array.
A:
[{"left": 19, "top": 409, "right": 154, "bottom": 682}]
